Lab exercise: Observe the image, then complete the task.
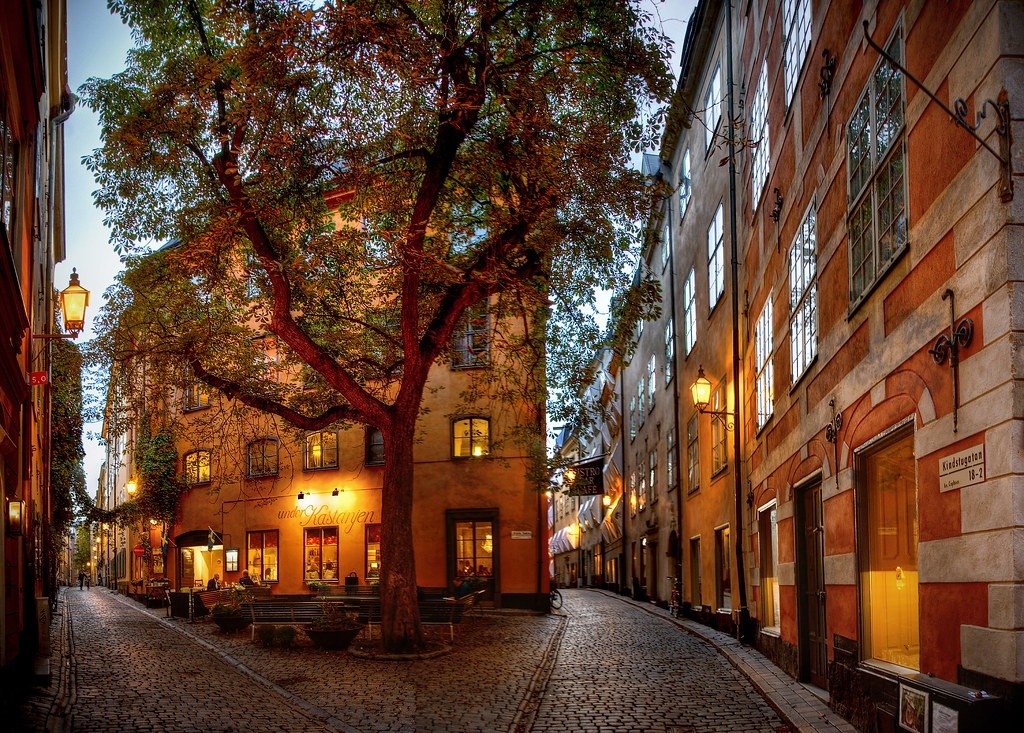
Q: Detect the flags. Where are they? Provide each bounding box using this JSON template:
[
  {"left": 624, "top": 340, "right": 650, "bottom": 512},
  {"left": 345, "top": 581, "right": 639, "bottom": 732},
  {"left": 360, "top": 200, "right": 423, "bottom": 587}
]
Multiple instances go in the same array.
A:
[
  {"left": 207, "top": 529, "right": 215, "bottom": 552},
  {"left": 159, "top": 532, "right": 169, "bottom": 553}
]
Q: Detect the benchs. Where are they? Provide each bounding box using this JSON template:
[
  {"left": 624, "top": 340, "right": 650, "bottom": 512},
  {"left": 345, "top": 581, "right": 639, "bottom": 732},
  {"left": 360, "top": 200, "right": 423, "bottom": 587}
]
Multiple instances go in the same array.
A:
[{"left": 194, "top": 586, "right": 487, "bottom": 645}]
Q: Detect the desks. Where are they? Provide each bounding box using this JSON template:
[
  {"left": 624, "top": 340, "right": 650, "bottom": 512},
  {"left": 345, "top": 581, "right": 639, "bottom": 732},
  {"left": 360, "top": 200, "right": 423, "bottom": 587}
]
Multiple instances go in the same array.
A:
[{"left": 146, "top": 585, "right": 165, "bottom": 606}]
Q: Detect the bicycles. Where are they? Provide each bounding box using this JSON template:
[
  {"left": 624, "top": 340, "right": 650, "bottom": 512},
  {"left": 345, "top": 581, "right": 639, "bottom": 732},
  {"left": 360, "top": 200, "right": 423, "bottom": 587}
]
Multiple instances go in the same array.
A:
[
  {"left": 670, "top": 591, "right": 682, "bottom": 618},
  {"left": 549, "top": 573, "right": 563, "bottom": 609}
]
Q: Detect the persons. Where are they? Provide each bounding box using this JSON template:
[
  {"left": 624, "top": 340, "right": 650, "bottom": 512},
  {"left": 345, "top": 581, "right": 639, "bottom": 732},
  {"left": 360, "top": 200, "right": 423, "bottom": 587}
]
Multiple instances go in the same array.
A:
[
  {"left": 76, "top": 568, "right": 91, "bottom": 590},
  {"left": 207, "top": 573, "right": 220, "bottom": 592},
  {"left": 240, "top": 570, "right": 252, "bottom": 586},
  {"left": 310, "top": 556, "right": 319, "bottom": 571},
  {"left": 443, "top": 560, "right": 491, "bottom": 610}
]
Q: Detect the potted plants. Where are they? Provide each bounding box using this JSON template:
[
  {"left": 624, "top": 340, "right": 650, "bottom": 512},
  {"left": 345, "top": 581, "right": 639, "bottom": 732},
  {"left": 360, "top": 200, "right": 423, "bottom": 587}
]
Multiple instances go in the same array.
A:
[
  {"left": 305, "top": 581, "right": 322, "bottom": 594},
  {"left": 254, "top": 624, "right": 276, "bottom": 647},
  {"left": 274, "top": 626, "right": 299, "bottom": 648},
  {"left": 208, "top": 603, "right": 244, "bottom": 632},
  {"left": 300, "top": 600, "right": 366, "bottom": 650}
]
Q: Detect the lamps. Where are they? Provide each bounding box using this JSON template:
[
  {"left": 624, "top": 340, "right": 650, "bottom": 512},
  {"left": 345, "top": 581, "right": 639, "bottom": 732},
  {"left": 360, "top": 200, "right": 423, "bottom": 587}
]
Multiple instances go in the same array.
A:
[
  {"left": 332, "top": 488, "right": 345, "bottom": 502},
  {"left": 603, "top": 490, "right": 613, "bottom": 509},
  {"left": 127, "top": 474, "right": 138, "bottom": 498},
  {"left": 7, "top": 497, "right": 26, "bottom": 537},
  {"left": 33, "top": 267, "right": 91, "bottom": 339},
  {"left": 564, "top": 464, "right": 576, "bottom": 485},
  {"left": 298, "top": 492, "right": 310, "bottom": 507},
  {"left": 688, "top": 364, "right": 738, "bottom": 431}
]
